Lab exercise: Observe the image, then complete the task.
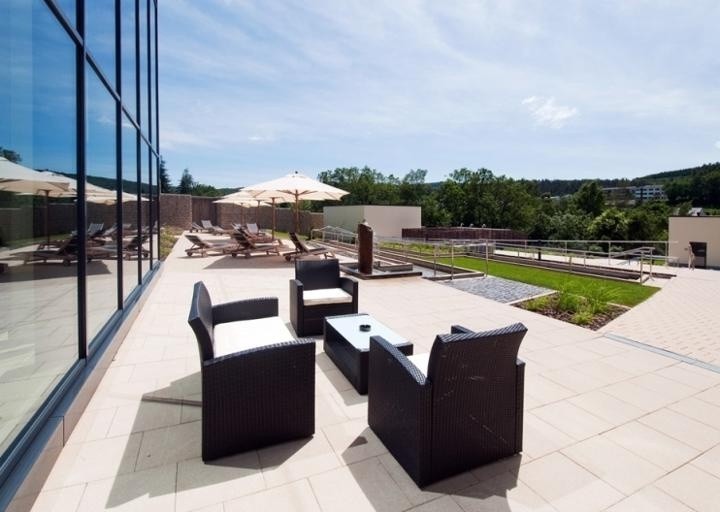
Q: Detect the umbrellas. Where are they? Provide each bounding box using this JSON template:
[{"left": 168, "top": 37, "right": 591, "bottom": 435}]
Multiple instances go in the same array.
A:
[
  {"left": 239, "top": 172, "right": 351, "bottom": 241},
  {"left": 0, "top": 156, "right": 70, "bottom": 202},
  {"left": 211, "top": 195, "right": 272, "bottom": 235},
  {"left": 14, "top": 170, "right": 114, "bottom": 249},
  {"left": 220, "top": 185, "right": 297, "bottom": 240},
  {"left": 77, "top": 181, "right": 156, "bottom": 208}
]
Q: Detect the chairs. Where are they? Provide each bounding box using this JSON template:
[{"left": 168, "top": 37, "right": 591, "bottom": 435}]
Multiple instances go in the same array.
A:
[
  {"left": 6, "top": 222, "right": 151, "bottom": 263},
  {"left": 186, "top": 220, "right": 281, "bottom": 258},
  {"left": 290, "top": 259, "right": 358, "bottom": 335},
  {"left": 282, "top": 233, "right": 329, "bottom": 264},
  {"left": 369, "top": 322, "right": 528, "bottom": 489}
]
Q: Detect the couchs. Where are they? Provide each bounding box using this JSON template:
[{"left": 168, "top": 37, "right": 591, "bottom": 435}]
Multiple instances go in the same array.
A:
[{"left": 187, "top": 281, "right": 315, "bottom": 462}]
